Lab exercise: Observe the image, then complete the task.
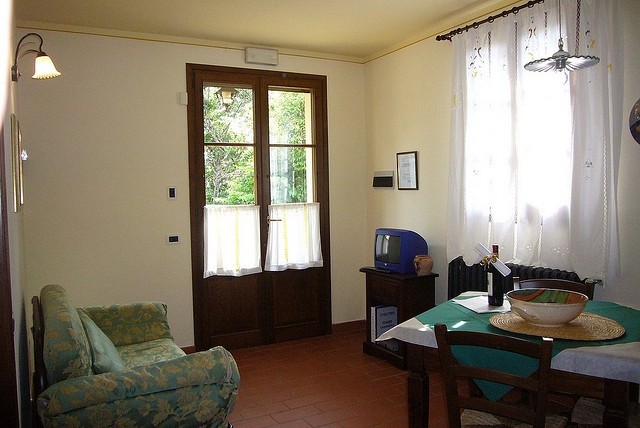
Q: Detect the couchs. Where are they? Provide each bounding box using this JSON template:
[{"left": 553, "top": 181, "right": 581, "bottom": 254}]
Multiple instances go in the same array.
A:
[{"left": 32, "top": 284, "right": 241, "bottom": 428}]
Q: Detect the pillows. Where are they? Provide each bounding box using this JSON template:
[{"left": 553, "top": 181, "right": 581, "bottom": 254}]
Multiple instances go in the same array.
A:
[{"left": 77, "top": 308, "right": 125, "bottom": 373}]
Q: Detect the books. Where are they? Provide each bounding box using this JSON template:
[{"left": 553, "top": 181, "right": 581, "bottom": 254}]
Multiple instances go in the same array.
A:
[{"left": 370, "top": 305, "right": 398, "bottom": 344}]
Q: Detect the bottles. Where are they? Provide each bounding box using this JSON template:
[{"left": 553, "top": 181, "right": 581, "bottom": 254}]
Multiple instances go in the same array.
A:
[{"left": 486, "top": 244, "right": 503, "bottom": 306}]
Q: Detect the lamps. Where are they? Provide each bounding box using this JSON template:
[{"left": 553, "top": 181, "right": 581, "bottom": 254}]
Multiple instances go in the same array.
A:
[
  {"left": 523, "top": 0, "right": 600, "bottom": 72},
  {"left": 213, "top": 86, "right": 240, "bottom": 111},
  {"left": 11, "top": 32, "right": 61, "bottom": 80}
]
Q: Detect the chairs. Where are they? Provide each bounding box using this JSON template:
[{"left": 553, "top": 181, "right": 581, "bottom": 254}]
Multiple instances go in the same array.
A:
[
  {"left": 571, "top": 396, "right": 640, "bottom": 427},
  {"left": 436, "top": 323, "right": 570, "bottom": 427}
]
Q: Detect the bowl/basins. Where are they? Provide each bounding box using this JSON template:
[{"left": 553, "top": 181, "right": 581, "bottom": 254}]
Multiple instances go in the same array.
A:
[{"left": 507, "top": 288, "right": 589, "bottom": 327}]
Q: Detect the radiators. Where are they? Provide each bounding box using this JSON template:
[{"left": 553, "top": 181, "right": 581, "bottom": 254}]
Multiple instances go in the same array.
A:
[{"left": 447, "top": 255, "right": 603, "bottom": 302}]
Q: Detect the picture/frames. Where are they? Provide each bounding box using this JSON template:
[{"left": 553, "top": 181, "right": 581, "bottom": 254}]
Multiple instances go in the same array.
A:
[
  {"left": 396, "top": 151, "right": 418, "bottom": 190},
  {"left": 11, "top": 114, "right": 24, "bottom": 213}
]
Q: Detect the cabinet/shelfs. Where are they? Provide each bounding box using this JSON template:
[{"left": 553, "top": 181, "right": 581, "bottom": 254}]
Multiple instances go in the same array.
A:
[{"left": 359, "top": 266, "right": 439, "bottom": 371}]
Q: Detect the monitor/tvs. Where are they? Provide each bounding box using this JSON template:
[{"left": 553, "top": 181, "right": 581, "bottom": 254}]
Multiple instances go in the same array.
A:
[{"left": 373, "top": 228, "right": 428, "bottom": 274}]
